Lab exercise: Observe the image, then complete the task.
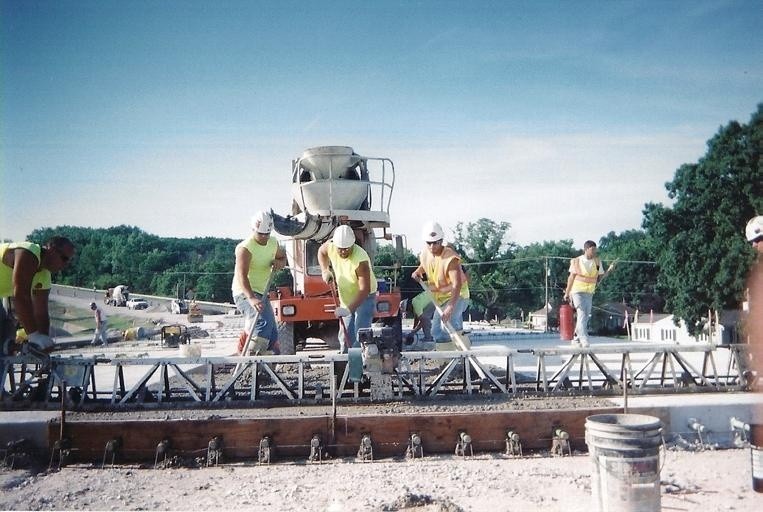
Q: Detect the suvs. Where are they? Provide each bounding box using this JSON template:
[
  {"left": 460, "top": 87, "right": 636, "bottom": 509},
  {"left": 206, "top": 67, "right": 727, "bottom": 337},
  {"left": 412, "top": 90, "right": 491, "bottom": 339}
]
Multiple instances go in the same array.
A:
[
  {"left": 171, "top": 299, "right": 188, "bottom": 314},
  {"left": 129, "top": 298, "right": 148, "bottom": 309}
]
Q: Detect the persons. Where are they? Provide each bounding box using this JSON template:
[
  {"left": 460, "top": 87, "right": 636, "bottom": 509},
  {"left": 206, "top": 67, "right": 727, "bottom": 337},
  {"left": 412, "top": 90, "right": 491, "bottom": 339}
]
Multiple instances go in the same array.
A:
[
  {"left": 90, "top": 302, "right": 110, "bottom": 347},
  {"left": 412, "top": 223, "right": 470, "bottom": 371},
  {"left": 566, "top": 240, "right": 598, "bottom": 349},
  {"left": 317, "top": 223, "right": 379, "bottom": 353},
  {"left": 399, "top": 291, "right": 435, "bottom": 342},
  {"left": 0, "top": 236, "right": 76, "bottom": 398},
  {"left": 745, "top": 214, "right": 763, "bottom": 260},
  {"left": 121, "top": 327, "right": 161, "bottom": 341},
  {"left": 231, "top": 210, "right": 287, "bottom": 386}
]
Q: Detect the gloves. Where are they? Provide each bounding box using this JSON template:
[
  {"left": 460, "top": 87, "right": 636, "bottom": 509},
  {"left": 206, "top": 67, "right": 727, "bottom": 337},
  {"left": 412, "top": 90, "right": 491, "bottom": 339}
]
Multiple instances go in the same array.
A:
[
  {"left": 322, "top": 270, "right": 334, "bottom": 284},
  {"left": 334, "top": 306, "right": 350, "bottom": 318},
  {"left": 27, "top": 329, "right": 55, "bottom": 350}
]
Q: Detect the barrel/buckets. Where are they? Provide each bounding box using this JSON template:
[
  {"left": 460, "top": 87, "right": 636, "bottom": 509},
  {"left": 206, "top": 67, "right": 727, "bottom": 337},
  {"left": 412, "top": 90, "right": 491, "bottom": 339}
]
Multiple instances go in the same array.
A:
[{"left": 584, "top": 413, "right": 667, "bottom": 511}]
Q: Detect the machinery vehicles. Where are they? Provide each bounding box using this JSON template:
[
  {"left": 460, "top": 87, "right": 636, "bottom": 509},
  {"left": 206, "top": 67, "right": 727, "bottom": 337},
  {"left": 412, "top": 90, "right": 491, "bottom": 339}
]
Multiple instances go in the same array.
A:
[
  {"left": 187, "top": 300, "right": 203, "bottom": 323},
  {"left": 104, "top": 285, "right": 131, "bottom": 306},
  {"left": 269, "top": 145, "right": 408, "bottom": 355}
]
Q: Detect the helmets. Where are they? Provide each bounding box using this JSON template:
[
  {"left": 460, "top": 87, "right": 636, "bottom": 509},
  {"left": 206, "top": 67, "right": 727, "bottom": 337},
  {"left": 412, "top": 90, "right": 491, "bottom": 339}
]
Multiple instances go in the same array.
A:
[
  {"left": 746, "top": 216, "right": 763, "bottom": 242},
  {"left": 253, "top": 212, "right": 272, "bottom": 233},
  {"left": 422, "top": 222, "right": 444, "bottom": 242},
  {"left": 399, "top": 298, "right": 407, "bottom": 312},
  {"left": 333, "top": 225, "right": 355, "bottom": 248}
]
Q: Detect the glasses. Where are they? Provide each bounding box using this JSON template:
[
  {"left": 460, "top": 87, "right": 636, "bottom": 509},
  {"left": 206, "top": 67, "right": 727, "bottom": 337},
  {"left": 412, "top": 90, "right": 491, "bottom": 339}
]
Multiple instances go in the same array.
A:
[
  {"left": 426, "top": 241, "right": 439, "bottom": 244},
  {"left": 749, "top": 236, "right": 763, "bottom": 245},
  {"left": 62, "top": 255, "right": 69, "bottom": 264}
]
